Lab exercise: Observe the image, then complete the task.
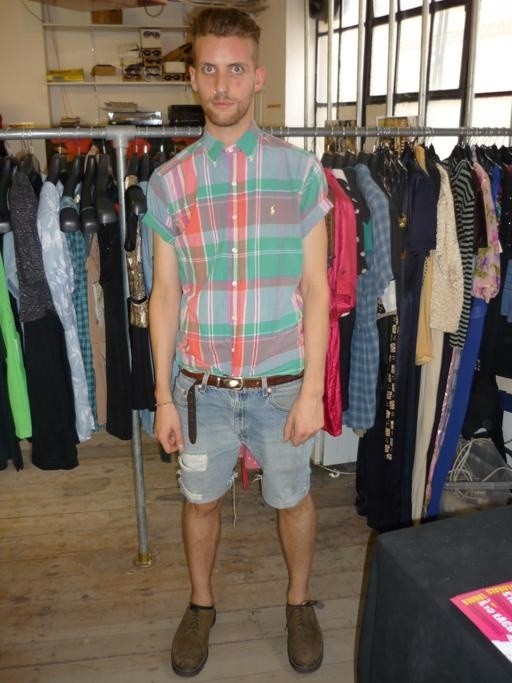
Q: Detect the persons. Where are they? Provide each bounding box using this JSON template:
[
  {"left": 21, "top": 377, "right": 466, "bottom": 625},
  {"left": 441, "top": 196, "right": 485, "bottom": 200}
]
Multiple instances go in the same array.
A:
[{"left": 140, "top": 4, "right": 336, "bottom": 681}]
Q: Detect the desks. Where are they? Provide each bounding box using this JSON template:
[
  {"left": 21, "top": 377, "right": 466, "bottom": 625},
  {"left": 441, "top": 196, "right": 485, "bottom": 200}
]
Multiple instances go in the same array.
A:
[{"left": 354, "top": 500, "right": 512, "bottom": 683}]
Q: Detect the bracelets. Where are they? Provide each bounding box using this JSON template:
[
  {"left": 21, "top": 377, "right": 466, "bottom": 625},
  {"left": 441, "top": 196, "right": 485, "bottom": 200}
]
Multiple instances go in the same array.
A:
[{"left": 155, "top": 400, "right": 176, "bottom": 408}]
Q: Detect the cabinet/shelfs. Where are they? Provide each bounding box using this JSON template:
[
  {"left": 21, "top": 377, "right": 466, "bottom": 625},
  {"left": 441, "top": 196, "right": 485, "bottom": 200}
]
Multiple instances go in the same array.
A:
[{"left": 41, "top": 0, "right": 256, "bottom": 172}]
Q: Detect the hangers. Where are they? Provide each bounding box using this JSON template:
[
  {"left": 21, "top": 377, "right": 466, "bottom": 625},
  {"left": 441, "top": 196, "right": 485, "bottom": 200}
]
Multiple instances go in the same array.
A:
[{"left": 1, "top": 122, "right": 512, "bottom": 235}]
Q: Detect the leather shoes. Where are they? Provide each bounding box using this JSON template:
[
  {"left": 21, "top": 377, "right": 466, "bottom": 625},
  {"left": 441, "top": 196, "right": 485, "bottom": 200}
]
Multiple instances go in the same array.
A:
[
  {"left": 285, "top": 599, "right": 325, "bottom": 673},
  {"left": 171, "top": 602, "right": 216, "bottom": 678}
]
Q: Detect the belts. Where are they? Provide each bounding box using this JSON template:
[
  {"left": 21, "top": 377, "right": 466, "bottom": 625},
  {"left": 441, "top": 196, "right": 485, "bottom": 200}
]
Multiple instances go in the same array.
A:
[{"left": 176, "top": 368, "right": 306, "bottom": 446}]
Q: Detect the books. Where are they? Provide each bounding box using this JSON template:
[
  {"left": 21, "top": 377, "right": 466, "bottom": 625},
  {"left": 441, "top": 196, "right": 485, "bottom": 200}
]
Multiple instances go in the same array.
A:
[
  {"left": 105, "top": 99, "right": 138, "bottom": 113},
  {"left": 93, "top": 74, "right": 122, "bottom": 82},
  {"left": 89, "top": 64, "right": 116, "bottom": 75}
]
